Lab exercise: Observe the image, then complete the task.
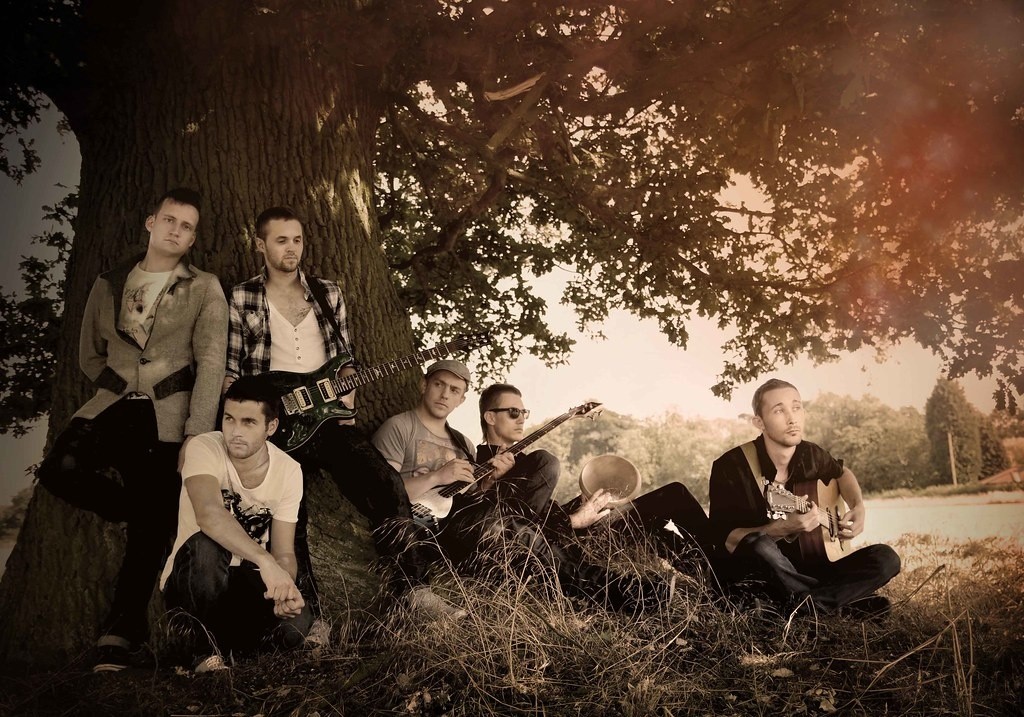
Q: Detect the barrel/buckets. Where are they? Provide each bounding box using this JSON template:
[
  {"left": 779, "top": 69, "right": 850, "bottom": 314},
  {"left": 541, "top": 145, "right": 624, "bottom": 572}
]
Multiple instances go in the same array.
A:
[{"left": 579, "top": 453, "right": 642, "bottom": 509}]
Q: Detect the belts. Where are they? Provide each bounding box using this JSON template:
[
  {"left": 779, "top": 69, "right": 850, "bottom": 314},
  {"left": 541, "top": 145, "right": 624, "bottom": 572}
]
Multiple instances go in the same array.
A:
[{"left": 124, "top": 392, "right": 151, "bottom": 399}]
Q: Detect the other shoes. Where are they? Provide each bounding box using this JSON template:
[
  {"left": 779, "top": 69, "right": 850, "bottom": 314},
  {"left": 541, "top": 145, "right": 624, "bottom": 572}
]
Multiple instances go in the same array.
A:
[
  {"left": 195, "top": 655, "right": 228, "bottom": 676},
  {"left": 842, "top": 594, "right": 891, "bottom": 624},
  {"left": 92, "top": 634, "right": 141, "bottom": 673}
]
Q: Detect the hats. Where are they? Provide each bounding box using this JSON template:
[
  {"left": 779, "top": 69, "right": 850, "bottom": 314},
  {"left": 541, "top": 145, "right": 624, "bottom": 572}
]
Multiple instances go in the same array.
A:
[{"left": 426, "top": 360, "right": 470, "bottom": 382}]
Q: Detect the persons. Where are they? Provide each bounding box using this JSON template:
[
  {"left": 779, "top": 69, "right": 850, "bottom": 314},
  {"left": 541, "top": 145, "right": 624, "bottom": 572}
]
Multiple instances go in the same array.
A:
[
  {"left": 706, "top": 377, "right": 901, "bottom": 627},
  {"left": 370, "top": 359, "right": 577, "bottom": 604},
  {"left": 475, "top": 381, "right": 709, "bottom": 573},
  {"left": 155, "top": 373, "right": 312, "bottom": 674},
  {"left": 220, "top": 205, "right": 471, "bottom": 657},
  {"left": 38, "top": 187, "right": 231, "bottom": 675}
]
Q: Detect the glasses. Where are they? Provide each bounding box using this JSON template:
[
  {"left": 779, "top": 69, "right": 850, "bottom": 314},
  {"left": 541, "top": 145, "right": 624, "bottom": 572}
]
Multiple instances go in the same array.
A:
[{"left": 488, "top": 407, "right": 530, "bottom": 419}]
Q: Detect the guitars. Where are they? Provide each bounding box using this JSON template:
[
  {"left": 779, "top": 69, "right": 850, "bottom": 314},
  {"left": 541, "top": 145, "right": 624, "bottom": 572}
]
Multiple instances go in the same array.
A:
[
  {"left": 253, "top": 328, "right": 493, "bottom": 455},
  {"left": 409, "top": 401, "right": 604, "bottom": 528},
  {"left": 762, "top": 477, "right": 851, "bottom": 568}
]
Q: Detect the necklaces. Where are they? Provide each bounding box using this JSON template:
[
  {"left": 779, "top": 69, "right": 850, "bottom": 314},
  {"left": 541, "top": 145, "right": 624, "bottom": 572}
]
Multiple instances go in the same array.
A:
[{"left": 774, "top": 462, "right": 796, "bottom": 484}]
